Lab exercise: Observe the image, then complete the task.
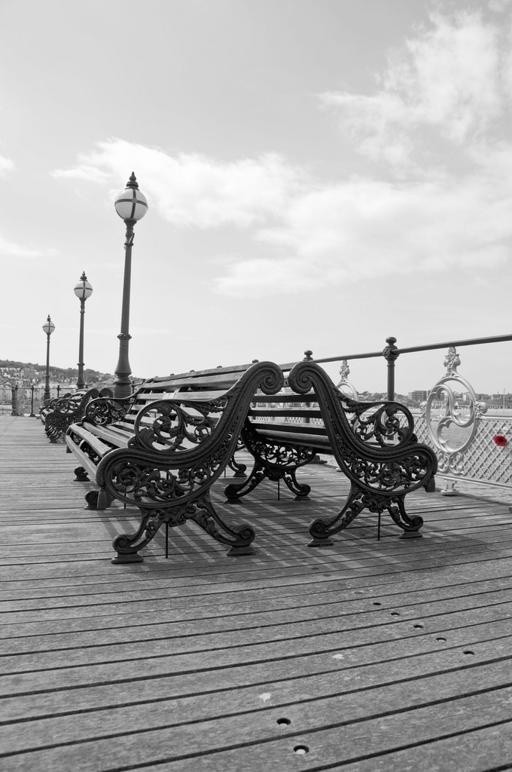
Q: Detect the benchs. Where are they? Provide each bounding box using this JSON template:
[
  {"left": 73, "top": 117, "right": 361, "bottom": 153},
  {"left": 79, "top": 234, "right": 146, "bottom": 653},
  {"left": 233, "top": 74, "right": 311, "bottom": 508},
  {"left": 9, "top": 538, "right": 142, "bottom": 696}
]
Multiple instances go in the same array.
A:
[{"left": 40, "top": 361, "right": 438, "bottom": 563}]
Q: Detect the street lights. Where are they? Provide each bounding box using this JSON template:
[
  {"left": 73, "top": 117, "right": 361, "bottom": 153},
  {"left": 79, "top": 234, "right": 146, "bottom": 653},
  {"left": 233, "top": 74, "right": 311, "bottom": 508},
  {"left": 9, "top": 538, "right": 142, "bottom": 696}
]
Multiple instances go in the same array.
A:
[
  {"left": 114, "top": 170, "right": 148, "bottom": 405},
  {"left": 42, "top": 314, "right": 55, "bottom": 399},
  {"left": 74, "top": 270, "right": 93, "bottom": 389}
]
